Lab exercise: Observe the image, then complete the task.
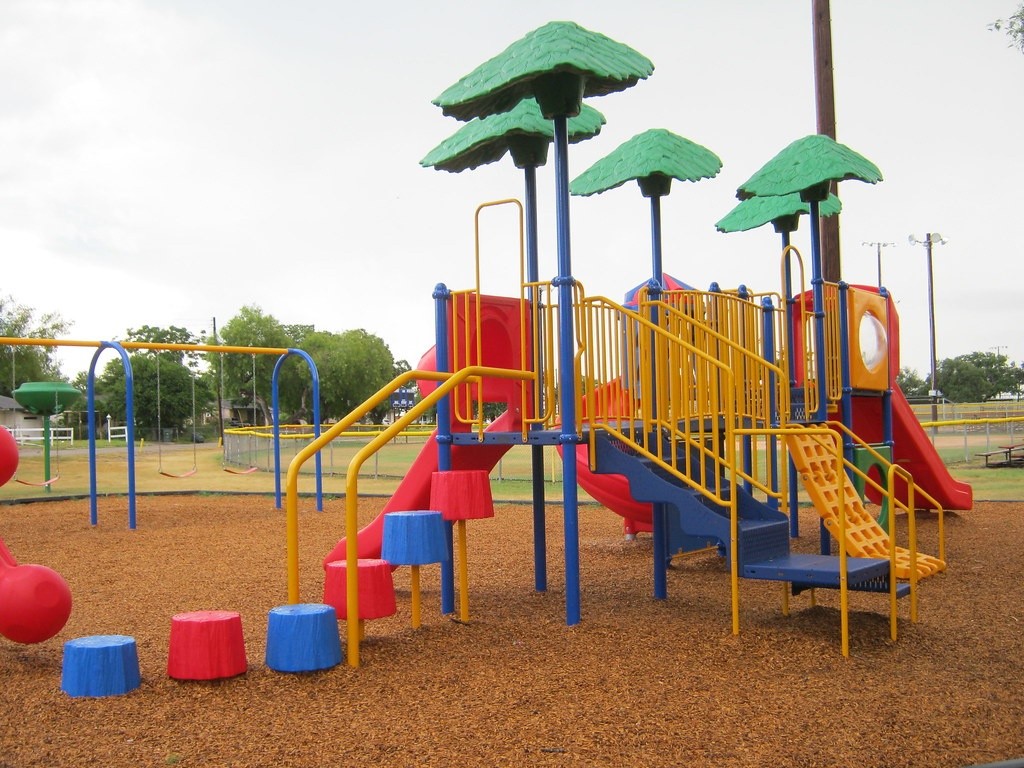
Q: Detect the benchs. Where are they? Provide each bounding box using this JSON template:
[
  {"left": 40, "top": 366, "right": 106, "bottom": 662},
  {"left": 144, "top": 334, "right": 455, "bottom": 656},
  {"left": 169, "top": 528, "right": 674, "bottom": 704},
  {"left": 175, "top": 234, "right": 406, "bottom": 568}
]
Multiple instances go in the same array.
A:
[{"left": 974, "top": 447, "right": 1024, "bottom": 467}]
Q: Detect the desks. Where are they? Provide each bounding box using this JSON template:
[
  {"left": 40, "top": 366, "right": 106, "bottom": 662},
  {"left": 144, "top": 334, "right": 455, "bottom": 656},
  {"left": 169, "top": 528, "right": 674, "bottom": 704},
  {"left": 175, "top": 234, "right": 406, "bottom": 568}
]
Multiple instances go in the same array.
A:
[{"left": 1000, "top": 442, "right": 1024, "bottom": 464}]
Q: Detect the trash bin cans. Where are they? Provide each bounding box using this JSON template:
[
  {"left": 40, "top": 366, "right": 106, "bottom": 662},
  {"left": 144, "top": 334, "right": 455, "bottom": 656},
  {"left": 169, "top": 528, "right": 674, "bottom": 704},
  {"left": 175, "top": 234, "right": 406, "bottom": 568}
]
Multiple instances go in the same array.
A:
[{"left": 162, "top": 427, "right": 173, "bottom": 443}]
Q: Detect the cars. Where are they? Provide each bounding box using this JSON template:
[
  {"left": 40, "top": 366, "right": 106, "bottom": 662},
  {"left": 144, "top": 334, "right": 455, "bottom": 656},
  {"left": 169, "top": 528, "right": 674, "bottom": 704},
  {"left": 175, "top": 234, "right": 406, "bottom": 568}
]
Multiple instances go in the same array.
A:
[{"left": 381, "top": 419, "right": 391, "bottom": 426}]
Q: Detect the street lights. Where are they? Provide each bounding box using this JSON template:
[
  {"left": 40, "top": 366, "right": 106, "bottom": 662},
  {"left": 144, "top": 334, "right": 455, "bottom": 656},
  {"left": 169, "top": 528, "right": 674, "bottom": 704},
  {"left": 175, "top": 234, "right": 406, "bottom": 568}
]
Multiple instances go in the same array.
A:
[
  {"left": 907, "top": 234, "right": 949, "bottom": 435},
  {"left": 861, "top": 241, "right": 897, "bottom": 289}
]
